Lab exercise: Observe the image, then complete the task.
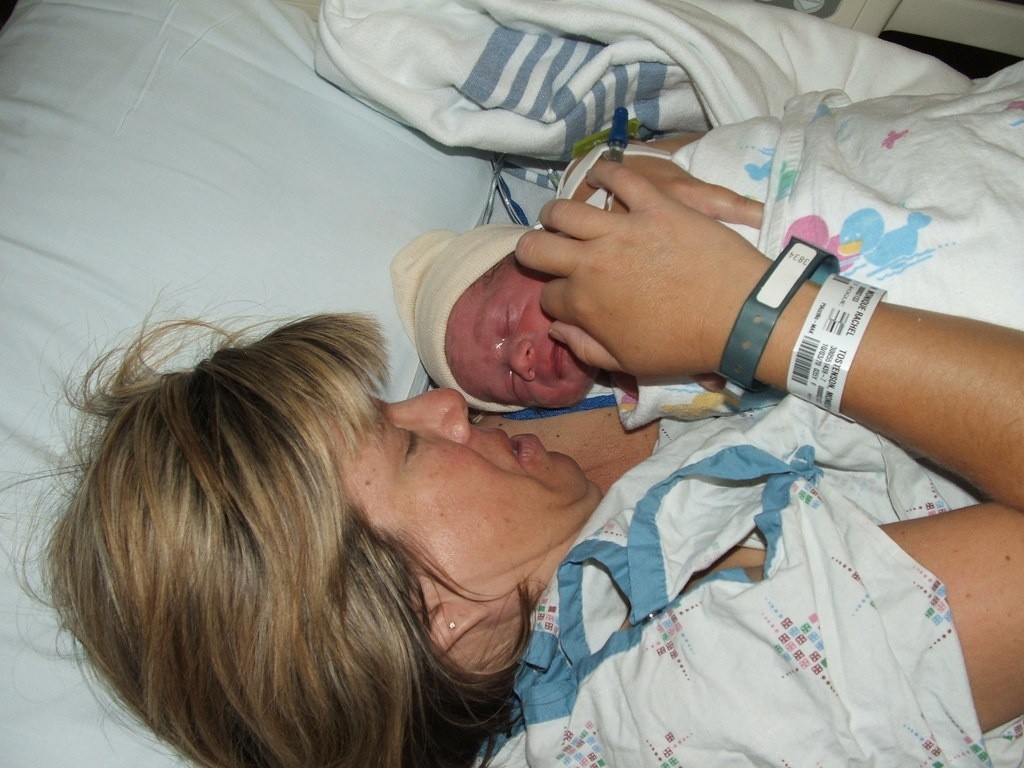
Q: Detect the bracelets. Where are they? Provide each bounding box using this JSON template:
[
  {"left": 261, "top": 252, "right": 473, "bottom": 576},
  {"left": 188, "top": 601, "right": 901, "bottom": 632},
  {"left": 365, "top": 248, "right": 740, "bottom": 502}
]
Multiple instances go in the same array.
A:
[
  {"left": 716, "top": 237, "right": 840, "bottom": 393},
  {"left": 787, "top": 272, "right": 887, "bottom": 423}
]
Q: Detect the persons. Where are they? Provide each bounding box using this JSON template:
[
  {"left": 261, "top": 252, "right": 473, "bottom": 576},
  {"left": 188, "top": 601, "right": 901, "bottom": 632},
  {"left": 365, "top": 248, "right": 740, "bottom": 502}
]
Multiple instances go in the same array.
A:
[
  {"left": 390, "top": 223, "right": 598, "bottom": 411},
  {"left": 43, "top": 131, "right": 1024, "bottom": 768}
]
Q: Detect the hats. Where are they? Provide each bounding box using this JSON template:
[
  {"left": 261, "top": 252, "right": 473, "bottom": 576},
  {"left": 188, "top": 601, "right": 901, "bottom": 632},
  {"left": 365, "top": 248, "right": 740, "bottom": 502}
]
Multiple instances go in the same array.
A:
[{"left": 393, "top": 224, "right": 539, "bottom": 412}]
